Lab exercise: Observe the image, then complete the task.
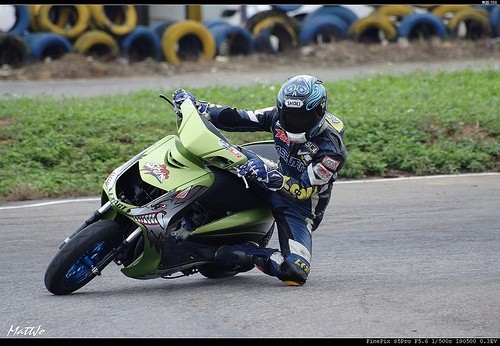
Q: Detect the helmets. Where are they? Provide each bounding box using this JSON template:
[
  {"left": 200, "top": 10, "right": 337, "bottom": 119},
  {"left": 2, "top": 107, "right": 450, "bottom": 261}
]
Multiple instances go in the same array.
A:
[{"left": 277, "top": 74, "right": 326, "bottom": 133}]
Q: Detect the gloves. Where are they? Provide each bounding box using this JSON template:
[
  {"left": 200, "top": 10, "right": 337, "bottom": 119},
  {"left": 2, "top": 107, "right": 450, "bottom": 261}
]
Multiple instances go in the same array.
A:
[
  {"left": 173, "top": 88, "right": 212, "bottom": 119},
  {"left": 236, "top": 146, "right": 270, "bottom": 182}
]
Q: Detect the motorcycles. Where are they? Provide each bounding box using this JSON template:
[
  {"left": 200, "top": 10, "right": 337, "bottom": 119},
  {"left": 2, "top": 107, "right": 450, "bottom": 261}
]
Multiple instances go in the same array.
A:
[{"left": 43, "top": 89, "right": 294, "bottom": 293}]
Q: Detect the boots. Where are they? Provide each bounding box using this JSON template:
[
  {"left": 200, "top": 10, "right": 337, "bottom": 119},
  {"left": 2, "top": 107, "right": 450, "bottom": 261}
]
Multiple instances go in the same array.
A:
[{"left": 214, "top": 242, "right": 278, "bottom": 276}]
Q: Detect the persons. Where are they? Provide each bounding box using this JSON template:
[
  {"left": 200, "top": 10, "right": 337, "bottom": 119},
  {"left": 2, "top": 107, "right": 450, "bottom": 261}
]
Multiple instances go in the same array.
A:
[{"left": 173, "top": 75, "right": 348, "bottom": 286}]
